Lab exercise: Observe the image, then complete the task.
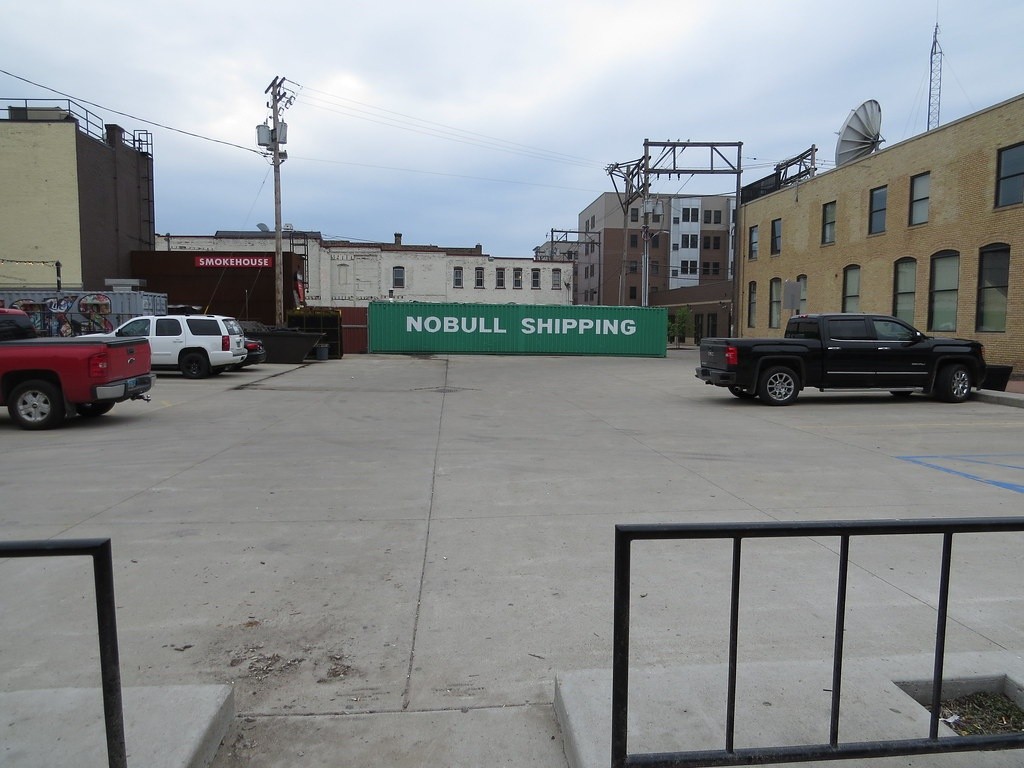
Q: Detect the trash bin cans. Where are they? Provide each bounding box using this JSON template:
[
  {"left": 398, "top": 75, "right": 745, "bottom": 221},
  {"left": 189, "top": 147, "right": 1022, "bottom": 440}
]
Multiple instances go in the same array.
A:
[{"left": 314, "top": 343, "right": 330, "bottom": 361}]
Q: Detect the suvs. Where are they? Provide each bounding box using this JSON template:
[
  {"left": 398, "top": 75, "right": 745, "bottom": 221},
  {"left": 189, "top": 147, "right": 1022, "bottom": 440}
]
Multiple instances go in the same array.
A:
[{"left": 75, "top": 313, "right": 247, "bottom": 379}]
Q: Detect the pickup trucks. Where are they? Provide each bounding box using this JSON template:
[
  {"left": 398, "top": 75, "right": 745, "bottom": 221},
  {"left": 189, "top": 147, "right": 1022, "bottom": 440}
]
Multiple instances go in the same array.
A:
[
  {"left": 0, "top": 308, "right": 154, "bottom": 432},
  {"left": 695, "top": 314, "right": 990, "bottom": 408}
]
